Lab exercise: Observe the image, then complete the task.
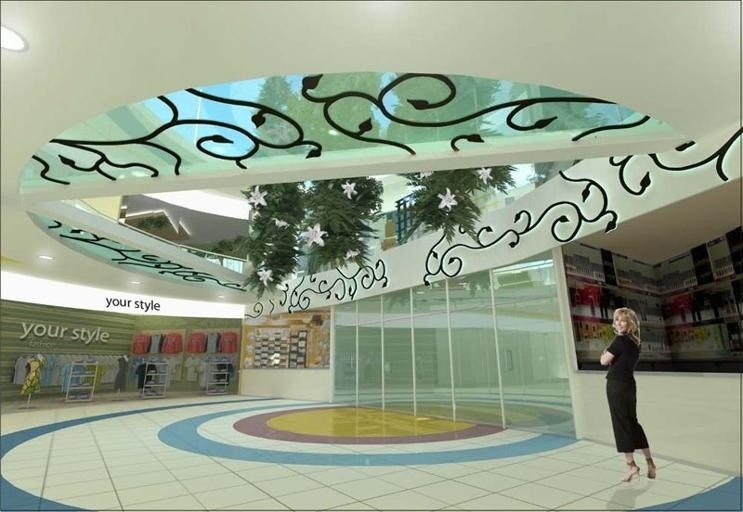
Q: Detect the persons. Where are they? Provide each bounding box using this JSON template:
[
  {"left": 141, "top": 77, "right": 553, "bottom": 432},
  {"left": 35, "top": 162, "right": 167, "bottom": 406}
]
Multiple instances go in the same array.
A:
[{"left": 600, "top": 308, "right": 656, "bottom": 482}]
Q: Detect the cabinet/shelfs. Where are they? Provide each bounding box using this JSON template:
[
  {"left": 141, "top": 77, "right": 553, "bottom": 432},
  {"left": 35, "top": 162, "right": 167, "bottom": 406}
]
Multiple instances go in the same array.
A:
[
  {"left": 65, "top": 361, "right": 98, "bottom": 403},
  {"left": 138, "top": 362, "right": 169, "bottom": 399},
  {"left": 205, "top": 362, "right": 229, "bottom": 395}
]
